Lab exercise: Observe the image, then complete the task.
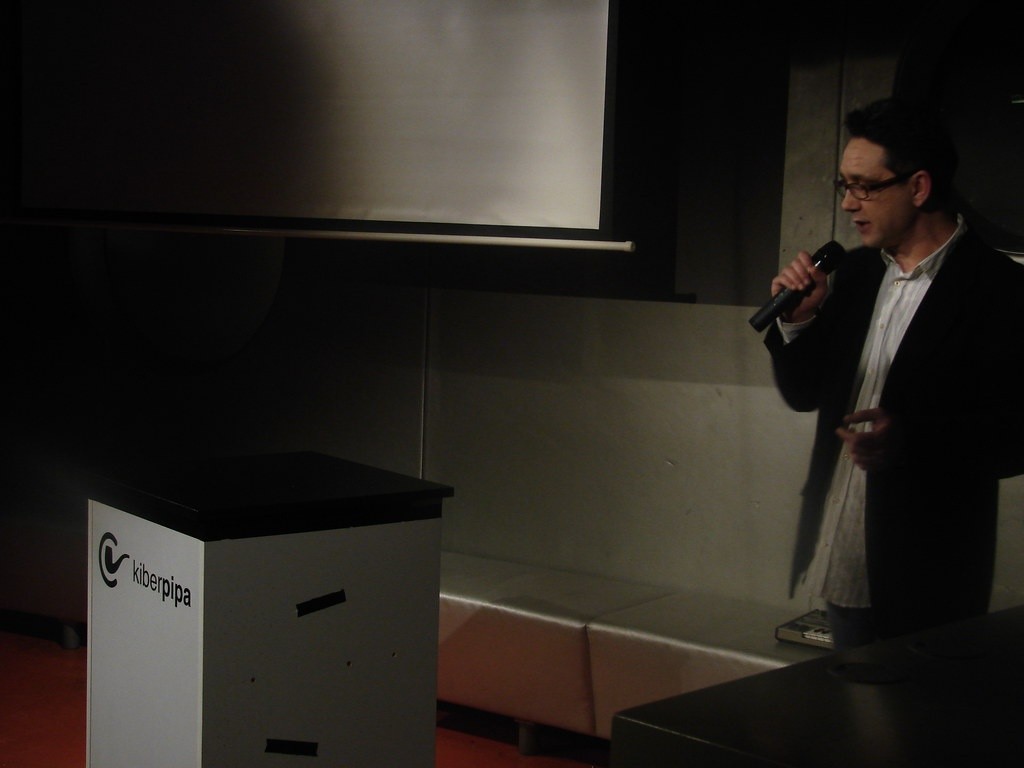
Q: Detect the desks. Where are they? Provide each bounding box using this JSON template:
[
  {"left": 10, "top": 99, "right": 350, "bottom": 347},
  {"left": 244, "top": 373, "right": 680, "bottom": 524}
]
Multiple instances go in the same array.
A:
[{"left": 615, "top": 604, "right": 1024, "bottom": 768}]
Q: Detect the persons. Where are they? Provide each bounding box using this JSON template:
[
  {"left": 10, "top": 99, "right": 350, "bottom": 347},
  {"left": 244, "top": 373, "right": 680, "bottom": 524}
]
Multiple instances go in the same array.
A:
[{"left": 760, "top": 88, "right": 1023, "bottom": 651}]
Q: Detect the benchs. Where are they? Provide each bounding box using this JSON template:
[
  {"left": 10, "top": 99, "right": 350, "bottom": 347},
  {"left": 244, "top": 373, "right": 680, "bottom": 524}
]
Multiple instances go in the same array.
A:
[
  {"left": 585, "top": 592, "right": 826, "bottom": 744},
  {"left": 439, "top": 548, "right": 680, "bottom": 756}
]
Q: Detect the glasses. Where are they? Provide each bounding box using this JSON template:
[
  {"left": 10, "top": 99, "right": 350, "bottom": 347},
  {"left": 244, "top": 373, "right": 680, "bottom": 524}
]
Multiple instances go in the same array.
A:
[{"left": 833, "top": 170, "right": 914, "bottom": 202}]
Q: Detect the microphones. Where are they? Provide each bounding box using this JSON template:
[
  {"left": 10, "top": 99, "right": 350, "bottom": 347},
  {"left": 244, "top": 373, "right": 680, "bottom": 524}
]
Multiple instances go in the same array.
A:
[{"left": 748, "top": 241, "right": 846, "bottom": 332}]
